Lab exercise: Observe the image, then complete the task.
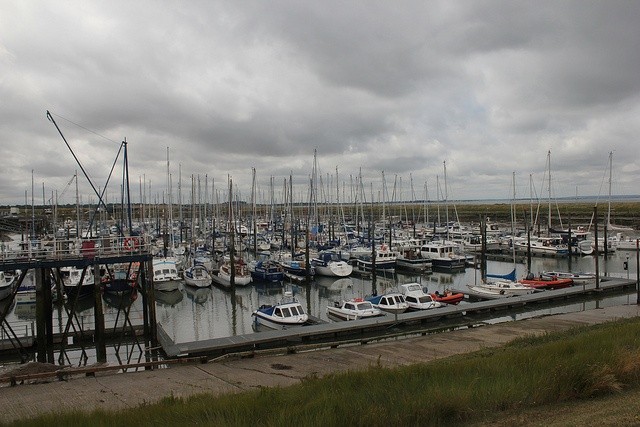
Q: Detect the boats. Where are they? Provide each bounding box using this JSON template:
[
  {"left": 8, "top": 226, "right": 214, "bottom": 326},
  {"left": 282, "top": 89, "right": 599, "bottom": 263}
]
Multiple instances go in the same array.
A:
[
  {"left": 250, "top": 287, "right": 312, "bottom": 330},
  {"left": 366, "top": 292, "right": 410, "bottom": 315},
  {"left": 1, "top": 251, "right": 17, "bottom": 301},
  {"left": 327, "top": 295, "right": 384, "bottom": 323}
]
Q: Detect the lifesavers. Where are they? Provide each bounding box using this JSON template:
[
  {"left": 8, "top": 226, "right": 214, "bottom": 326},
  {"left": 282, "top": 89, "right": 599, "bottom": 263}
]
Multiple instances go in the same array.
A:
[{"left": 124, "top": 236, "right": 139, "bottom": 250}]
